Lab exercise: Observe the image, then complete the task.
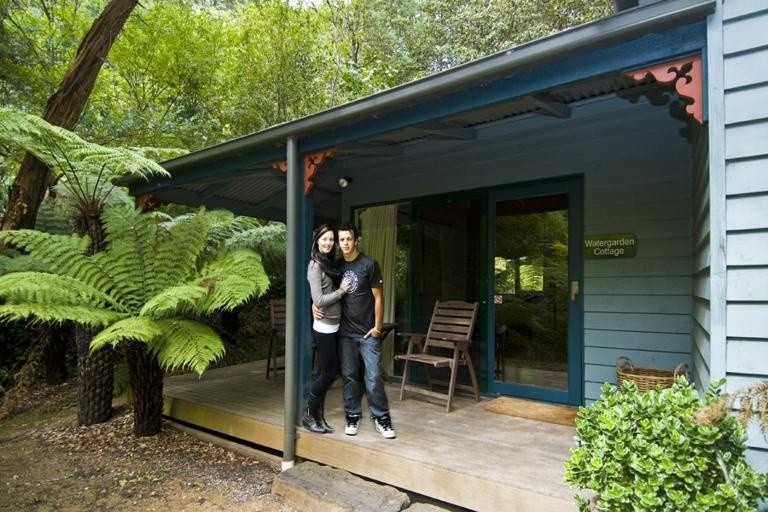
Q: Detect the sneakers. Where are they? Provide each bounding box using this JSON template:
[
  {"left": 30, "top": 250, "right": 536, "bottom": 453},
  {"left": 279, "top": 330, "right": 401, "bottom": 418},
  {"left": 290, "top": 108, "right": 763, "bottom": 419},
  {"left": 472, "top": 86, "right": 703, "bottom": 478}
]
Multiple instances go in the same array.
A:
[
  {"left": 375, "top": 418, "right": 396, "bottom": 439},
  {"left": 345, "top": 412, "right": 362, "bottom": 435}
]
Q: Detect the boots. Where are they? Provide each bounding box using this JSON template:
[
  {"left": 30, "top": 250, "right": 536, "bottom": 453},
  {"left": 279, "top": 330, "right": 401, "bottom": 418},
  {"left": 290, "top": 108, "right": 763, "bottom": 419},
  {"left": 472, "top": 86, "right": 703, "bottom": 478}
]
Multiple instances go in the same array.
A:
[{"left": 302, "top": 391, "right": 334, "bottom": 434}]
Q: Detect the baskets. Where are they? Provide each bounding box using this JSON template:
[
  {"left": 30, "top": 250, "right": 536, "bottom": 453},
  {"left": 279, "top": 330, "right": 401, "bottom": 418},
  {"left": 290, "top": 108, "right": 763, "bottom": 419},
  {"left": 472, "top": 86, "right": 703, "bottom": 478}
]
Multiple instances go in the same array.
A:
[{"left": 615, "top": 357, "right": 689, "bottom": 396}]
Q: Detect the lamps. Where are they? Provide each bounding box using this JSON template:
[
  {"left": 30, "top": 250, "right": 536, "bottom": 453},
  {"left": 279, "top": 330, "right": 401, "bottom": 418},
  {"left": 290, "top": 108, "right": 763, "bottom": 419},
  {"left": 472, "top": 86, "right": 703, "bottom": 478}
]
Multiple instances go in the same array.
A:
[{"left": 334, "top": 174, "right": 353, "bottom": 190}]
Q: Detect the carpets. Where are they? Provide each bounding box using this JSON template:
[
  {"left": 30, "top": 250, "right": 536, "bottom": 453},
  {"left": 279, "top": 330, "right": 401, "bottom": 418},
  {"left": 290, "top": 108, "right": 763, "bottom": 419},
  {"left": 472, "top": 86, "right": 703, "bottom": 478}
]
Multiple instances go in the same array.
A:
[{"left": 483, "top": 394, "right": 580, "bottom": 427}]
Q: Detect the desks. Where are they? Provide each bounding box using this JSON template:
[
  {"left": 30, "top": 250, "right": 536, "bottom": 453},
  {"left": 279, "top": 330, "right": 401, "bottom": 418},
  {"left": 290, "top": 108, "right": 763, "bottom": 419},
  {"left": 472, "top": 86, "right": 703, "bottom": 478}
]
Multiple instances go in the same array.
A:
[{"left": 380, "top": 321, "right": 401, "bottom": 384}]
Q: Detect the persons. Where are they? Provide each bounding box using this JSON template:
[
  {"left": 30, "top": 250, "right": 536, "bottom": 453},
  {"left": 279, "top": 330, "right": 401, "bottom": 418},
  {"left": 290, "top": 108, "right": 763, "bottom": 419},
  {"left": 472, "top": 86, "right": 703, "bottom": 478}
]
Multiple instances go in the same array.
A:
[
  {"left": 311, "top": 221, "right": 397, "bottom": 440},
  {"left": 300, "top": 222, "right": 350, "bottom": 435}
]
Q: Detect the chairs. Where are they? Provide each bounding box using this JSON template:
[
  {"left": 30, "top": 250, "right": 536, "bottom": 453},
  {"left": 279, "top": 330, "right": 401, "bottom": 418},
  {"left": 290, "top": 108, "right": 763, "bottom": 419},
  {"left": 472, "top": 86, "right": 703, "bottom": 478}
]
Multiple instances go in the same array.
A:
[
  {"left": 266, "top": 296, "right": 318, "bottom": 382},
  {"left": 393, "top": 297, "right": 481, "bottom": 413}
]
